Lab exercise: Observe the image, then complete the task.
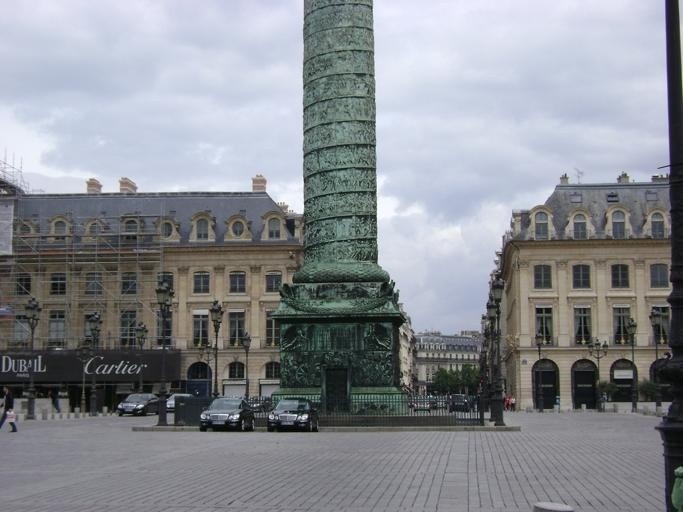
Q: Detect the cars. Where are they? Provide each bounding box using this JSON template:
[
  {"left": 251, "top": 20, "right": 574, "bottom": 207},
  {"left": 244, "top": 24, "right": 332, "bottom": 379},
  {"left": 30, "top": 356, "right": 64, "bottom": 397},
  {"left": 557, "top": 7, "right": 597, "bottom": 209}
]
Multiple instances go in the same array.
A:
[
  {"left": 199, "top": 395, "right": 253, "bottom": 432},
  {"left": 247, "top": 396, "right": 276, "bottom": 414},
  {"left": 165, "top": 394, "right": 193, "bottom": 411},
  {"left": 407, "top": 391, "right": 477, "bottom": 413},
  {"left": 269, "top": 397, "right": 320, "bottom": 434},
  {"left": 117, "top": 393, "right": 160, "bottom": 417}
]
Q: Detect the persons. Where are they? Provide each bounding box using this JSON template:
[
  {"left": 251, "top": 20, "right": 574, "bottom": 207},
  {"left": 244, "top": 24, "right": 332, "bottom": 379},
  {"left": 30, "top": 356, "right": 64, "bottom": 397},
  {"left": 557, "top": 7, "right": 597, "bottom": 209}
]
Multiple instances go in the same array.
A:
[
  {"left": 0, "top": 385, "right": 18, "bottom": 432},
  {"left": 502, "top": 391, "right": 516, "bottom": 416}
]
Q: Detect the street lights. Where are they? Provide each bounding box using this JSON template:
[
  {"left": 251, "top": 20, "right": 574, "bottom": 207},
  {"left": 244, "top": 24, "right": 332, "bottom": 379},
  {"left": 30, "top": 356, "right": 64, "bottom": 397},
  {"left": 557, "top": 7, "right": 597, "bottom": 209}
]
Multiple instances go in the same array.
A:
[
  {"left": 588, "top": 337, "right": 609, "bottom": 412},
  {"left": 23, "top": 297, "right": 41, "bottom": 420},
  {"left": 480, "top": 268, "right": 507, "bottom": 426},
  {"left": 155, "top": 279, "right": 175, "bottom": 423},
  {"left": 88, "top": 309, "right": 103, "bottom": 417},
  {"left": 199, "top": 339, "right": 217, "bottom": 366},
  {"left": 625, "top": 316, "right": 639, "bottom": 412},
  {"left": 77, "top": 336, "right": 91, "bottom": 412},
  {"left": 648, "top": 309, "right": 663, "bottom": 410},
  {"left": 205, "top": 299, "right": 225, "bottom": 395},
  {"left": 135, "top": 321, "right": 147, "bottom": 392},
  {"left": 535, "top": 331, "right": 542, "bottom": 413},
  {"left": 238, "top": 333, "right": 252, "bottom": 400}
]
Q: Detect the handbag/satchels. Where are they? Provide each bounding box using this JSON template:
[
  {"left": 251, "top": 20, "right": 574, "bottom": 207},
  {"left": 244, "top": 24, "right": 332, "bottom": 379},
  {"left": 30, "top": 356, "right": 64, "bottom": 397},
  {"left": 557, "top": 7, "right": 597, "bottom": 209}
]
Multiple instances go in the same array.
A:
[{"left": 6, "top": 411, "right": 16, "bottom": 422}]
